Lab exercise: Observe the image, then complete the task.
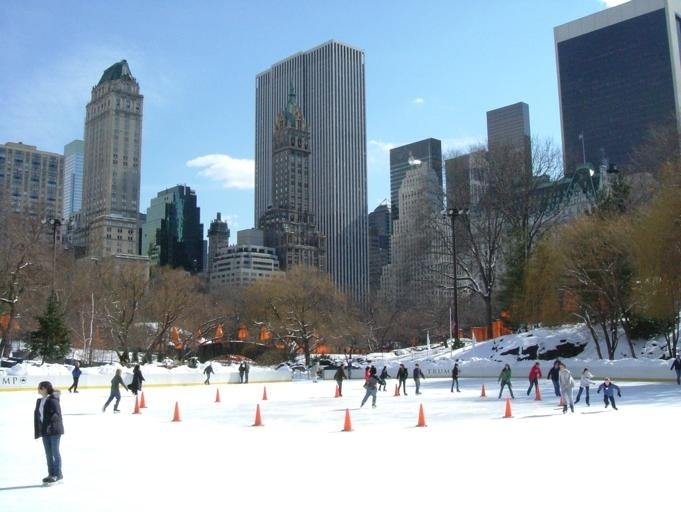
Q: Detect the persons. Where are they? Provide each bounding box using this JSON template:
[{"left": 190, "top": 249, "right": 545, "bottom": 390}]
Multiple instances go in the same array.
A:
[
  {"left": 204, "top": 364, "right": 215, "bottom": 385},
  {"left": 498, "top": 363, "right": 515, "bottom": 399},
  {"left": 574, "top": 367, "right": 597, "bottom": 407},
  {"left": 413, "top": 363, "right": 426, "bottom": 394},
  {"left": 365, "top": 359, "right": 373, "bottom": 382},
  {"left": 68, "top": 361, "right": 83, "bottom": 393},
  {"left": 527, "top": 361, "right": 543, "bottom": 396},
  {"left": 547, "top": 359, "right": 562, "bottom": 397},
  {"left": 451, "top": 362, "right": 462, "bottom": 392},
  {"left": 335, "top": 363, "right": 348, "bottom": 396},
  {"left": 244, "top": 361, "right": 250, "bottom": 383},
  {"left": 33, "top": 380, "right": 65, "bottom": 483},
  {"left": 128, "top": 364, "right": 146, "bottom": 395},
  {"left": 360, "top": 367, "right": 385, "bottom": 407},
  {"left": 397, "top": 364, "right": 409, "bottom": 395},
  {"left": 103, "top": 368, "right": 130, "bottom": 411},
  {"left": 558, "top": 362, "right": 576, "bottom": 413},
  {"left": 378, "top": 366, "right": 393, "bottom": 392},
  {"left": 670, "top": 354, "right": 681, "bottom": 385},
  {"left": 239, "top": 363, "right": 245, "bottom": 383},
  {"left": 598, "top": 376, "right": 622, "bottom": 410}
]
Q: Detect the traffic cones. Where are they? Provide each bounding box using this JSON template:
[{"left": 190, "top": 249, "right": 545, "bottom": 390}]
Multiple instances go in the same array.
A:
[
  {"left": 251, "top": 403, "right": 265, "bottom": 427},
  {"left": 534, "top": 384, "right": 541, "bottom": 401},
  {"left": 132, "top": 396, "right": 142, "bottom": 416},
  {"left": 502, "top": 399, "right": 514, "bottom": 419},
  {"left": 261, "top": 385, "right": 268, "bottom": 401},
  {"left": 333, "top": 384, "right": 340, "bottom": 399},
  {"left": 393, "top": 383, "right": 400, "bottom": 398},
  {"left": 415, "top": 403, "right": 427, "bottom": 428},
  {"left": 169, "top": 400, "right": 183, "bottom": 423},
  {"left": 479, "top": 384, "right": 487, "bottom": 399},
  {"left": 215, "top": 388, "right": 221, "bottom": 403},
  {"left": 341, "top": 407, "right": 356, "bottom": 433},
  {"left": 139, "top": 392, "right": 147, "bottom": 408},
  {"left": 559, "top": 394, "right": 564, "bottom": 407}
]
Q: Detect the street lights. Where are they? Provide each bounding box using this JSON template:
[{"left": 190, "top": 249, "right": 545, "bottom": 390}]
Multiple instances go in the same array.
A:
[
  {"left": 40, "top": 217, "right": 76, "bottom": 296},
  {"left": 440, "top": 206, "right": 470, "bottom": 344}
]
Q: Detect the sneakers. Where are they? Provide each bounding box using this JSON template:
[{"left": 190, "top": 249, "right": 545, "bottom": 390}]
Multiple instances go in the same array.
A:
[{"left": 43, "top": 473, "right": 63, "bottom": 482}]
